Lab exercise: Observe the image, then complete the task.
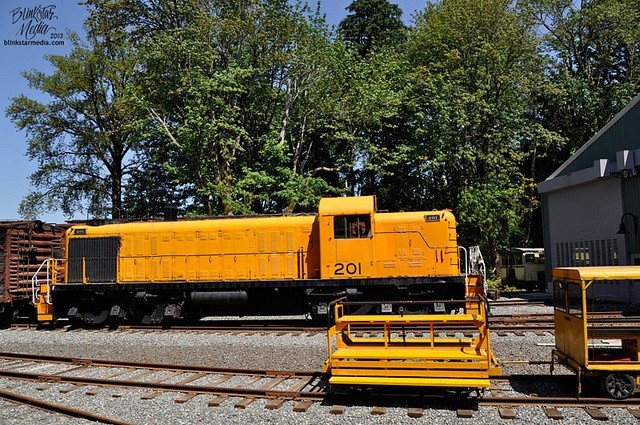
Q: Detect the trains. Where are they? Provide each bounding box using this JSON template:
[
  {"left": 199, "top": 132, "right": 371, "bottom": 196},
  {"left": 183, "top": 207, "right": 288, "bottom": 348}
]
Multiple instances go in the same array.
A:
[
  {"left": 323, "top": 266, "right": 640, "bottom": 400},
  {"left": 32, "top": 196, "right": 498, "bottom": 328},
  {"left": 499, "top": 247, "right": 617, "bottom": 292}
]
